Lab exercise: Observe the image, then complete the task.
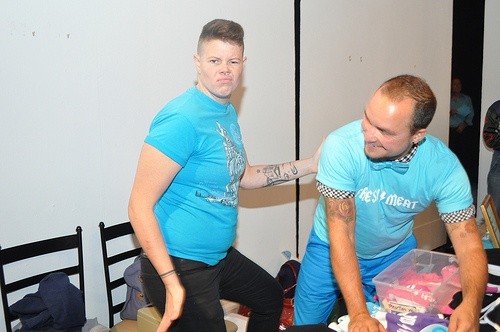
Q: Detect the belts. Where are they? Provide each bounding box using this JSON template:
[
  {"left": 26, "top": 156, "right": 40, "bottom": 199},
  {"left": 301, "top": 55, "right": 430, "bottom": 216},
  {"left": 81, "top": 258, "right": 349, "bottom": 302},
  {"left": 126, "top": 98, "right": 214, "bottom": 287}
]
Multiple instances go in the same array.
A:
[{"left": 141, "top": 259, "right": 208, "bottom": 279}]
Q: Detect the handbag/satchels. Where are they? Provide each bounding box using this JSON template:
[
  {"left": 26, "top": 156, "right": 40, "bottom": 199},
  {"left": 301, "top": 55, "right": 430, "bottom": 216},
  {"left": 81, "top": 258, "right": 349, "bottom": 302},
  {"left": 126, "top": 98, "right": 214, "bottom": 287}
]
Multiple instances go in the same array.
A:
[
  {"left": 121, "top": 253, "right": 149, "bottom": 319},
  {"left": 4, "top": 272, "right": 88, "bottom": 332},
  {"left": 238, "top": 261, "right": 299, "bottom": 328}
]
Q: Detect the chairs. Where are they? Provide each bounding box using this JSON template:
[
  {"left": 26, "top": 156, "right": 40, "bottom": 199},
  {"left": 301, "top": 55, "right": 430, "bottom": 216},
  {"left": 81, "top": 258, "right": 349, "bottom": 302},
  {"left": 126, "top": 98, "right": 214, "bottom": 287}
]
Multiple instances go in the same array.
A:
[
  {"left": 481, "top": 194, "right": 500, "bottom": 248},
  {"left": 0, "top": 225, "right": 111, "bottom": 332},
  {"left": 99, "top": 220, "right": 144, "bottom": 330}
]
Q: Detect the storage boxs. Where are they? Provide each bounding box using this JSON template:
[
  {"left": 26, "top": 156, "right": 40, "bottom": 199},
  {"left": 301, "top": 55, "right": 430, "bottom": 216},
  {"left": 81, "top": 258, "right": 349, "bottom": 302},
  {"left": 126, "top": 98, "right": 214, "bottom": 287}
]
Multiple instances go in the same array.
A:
[
  {"left": 137, "top": 305, "right": 162, "bottom": 332},
  {"left": 373, "top": 249, "right": 461, "bottom": 317}
]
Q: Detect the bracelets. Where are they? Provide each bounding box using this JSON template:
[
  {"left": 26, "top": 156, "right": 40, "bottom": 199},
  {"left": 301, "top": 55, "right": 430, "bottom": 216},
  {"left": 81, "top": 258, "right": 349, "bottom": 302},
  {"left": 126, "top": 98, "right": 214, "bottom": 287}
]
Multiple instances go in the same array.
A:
[{"left": 158, "top": 268, "right": 177, "bottom": 278}]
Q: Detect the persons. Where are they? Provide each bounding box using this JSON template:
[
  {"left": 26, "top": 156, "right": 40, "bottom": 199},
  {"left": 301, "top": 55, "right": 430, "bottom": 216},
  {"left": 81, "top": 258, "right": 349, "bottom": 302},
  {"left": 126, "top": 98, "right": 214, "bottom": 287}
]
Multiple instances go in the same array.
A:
[
  {"left": 126, "top": 18, "right": 328, "bottom": 332},
  {"left": 449, "top": 77, "right": 500, "bottom": 221},
  {"left": 291, "top": 74, "right": 488, "bottom": 332}
]
